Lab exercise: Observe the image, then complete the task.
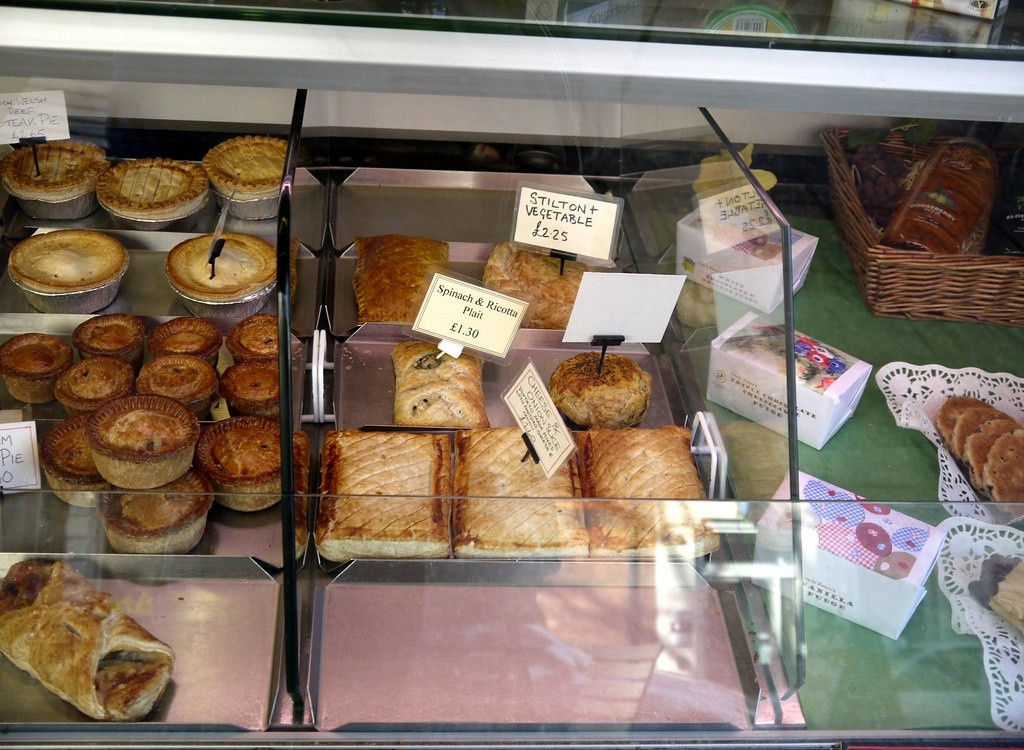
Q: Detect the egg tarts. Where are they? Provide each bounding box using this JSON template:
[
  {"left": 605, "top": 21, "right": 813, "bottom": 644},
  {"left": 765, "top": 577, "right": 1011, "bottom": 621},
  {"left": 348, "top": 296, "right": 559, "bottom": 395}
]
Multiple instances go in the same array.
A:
[{"left": 0, "top": 136, "right": 292, "bottom": 326}]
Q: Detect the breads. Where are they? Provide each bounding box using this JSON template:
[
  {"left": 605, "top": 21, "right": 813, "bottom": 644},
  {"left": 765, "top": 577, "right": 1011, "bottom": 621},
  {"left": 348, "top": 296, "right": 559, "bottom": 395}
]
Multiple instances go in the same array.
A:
[
  {"left": 0, "top": 236, "right": 722, "bottom": 561},
  {"left": 883, "top": 135, "right": 999, "bottom": 259}
]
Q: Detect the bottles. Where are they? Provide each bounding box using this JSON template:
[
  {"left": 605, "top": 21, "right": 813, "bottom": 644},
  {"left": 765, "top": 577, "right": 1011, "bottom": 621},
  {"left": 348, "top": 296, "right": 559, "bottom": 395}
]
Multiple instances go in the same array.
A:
[
  {"left": 882, "top": 120, "right": 1000, "bottom": 258},
  {"left": 982, "top": 133, "right": 1023, "bottom": 253}
]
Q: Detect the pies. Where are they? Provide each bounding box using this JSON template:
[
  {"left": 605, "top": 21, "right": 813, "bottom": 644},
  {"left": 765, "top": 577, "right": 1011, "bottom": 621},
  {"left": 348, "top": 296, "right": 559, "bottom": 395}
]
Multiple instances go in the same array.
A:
[
  {"left": 1, "top": 557, "right": 174, "bottom": 723},
  {"left": 934, "top": 396, "right": 1024, "bottom": 516}
]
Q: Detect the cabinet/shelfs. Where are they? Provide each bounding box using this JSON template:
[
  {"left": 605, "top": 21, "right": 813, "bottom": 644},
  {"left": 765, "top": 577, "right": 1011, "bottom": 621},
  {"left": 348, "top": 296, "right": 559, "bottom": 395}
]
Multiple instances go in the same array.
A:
[{"left": 0, "top": 0, "right": 1024, "bottom": 747}]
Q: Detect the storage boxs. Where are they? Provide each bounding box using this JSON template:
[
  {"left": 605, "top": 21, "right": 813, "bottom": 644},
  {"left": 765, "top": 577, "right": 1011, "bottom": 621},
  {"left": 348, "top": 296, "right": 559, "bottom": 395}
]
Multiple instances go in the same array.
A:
[{"left": 820, "top": 128, "right": 1023, "bottom": 326}]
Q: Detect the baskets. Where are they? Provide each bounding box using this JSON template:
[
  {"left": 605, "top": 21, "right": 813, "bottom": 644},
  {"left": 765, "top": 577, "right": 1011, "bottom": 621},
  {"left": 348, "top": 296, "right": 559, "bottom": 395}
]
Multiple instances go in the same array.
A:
[{"left": 816, "top": 127, "right": 1024, "bottom": 329}]
